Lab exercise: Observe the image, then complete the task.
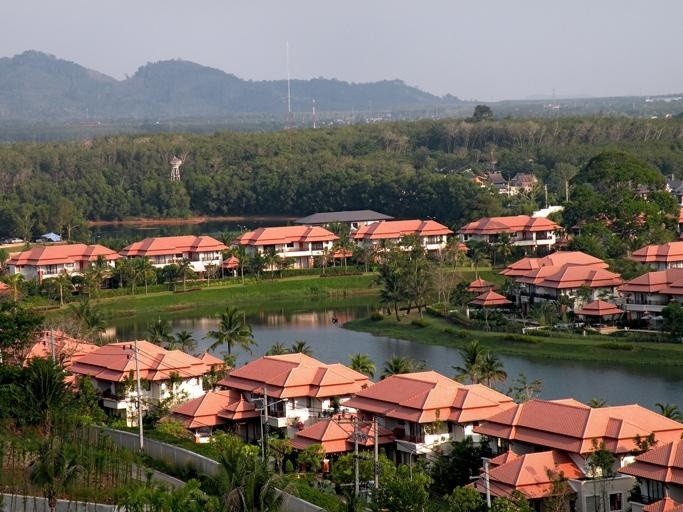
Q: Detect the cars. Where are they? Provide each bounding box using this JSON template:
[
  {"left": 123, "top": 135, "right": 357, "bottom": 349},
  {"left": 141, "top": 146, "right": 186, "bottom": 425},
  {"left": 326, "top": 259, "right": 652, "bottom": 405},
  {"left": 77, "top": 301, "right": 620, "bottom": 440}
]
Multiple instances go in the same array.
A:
[{"left": 5, "top": 238, "right": 26, "bottom": 244}]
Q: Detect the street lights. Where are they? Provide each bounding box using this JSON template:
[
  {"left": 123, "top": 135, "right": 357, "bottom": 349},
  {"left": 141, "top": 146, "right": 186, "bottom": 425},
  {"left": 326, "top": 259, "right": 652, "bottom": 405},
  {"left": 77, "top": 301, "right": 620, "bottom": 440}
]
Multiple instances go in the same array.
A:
[
  {"left": 65, "top": 223, "right": 81, "bottom": 241},
  {"left": 426, "top": 215, "right": 436, "bottom": 221},
  {"left": 263, "top": 388, "right": 290, "bottom": 463},
  {"left": 237, "top": 224, "right": 246, "bottom": 234}
]
